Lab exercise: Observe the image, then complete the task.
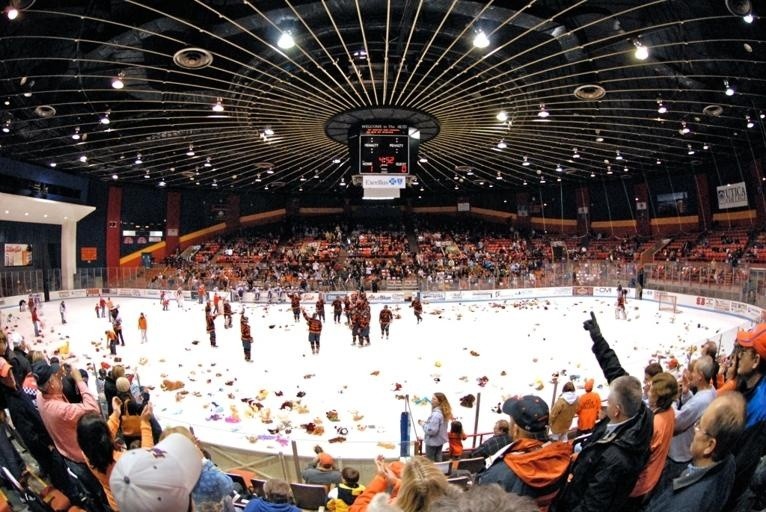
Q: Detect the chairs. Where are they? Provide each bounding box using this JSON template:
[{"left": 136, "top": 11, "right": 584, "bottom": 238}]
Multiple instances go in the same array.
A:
[
  {"left": 429, "top": 456, "right": 487, "bottom": 492},
  {"left": 224, "top": 473, "right": 327, "bottom": 511}
]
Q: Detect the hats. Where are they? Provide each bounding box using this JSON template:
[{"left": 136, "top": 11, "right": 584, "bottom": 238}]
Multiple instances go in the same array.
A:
[
  {"left": 109, "top": 432, "right": 234, "bottom": 512},
  {"left": 737, "top": 323, "right": 766, "bottom": 358},
  {"left": 0, "top": 357, "right": 13, "bottom": 378},
  {"left": 116, "top": 377, "right": 130, "bottom": 392},
  {"left": 503, "top": 395, "right": 548, "bottom": 432},
  {"left": 320, "top": 453, "right": 333, "bottom": 465},
  {"left": 33, "top": 360, "right": 59, "bottom": 385},
  {"left": 585, "top": 379, "right": 593, "bottom": 390}
]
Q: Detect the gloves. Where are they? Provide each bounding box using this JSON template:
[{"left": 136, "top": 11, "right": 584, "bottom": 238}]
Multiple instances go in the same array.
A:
[{"left": 583, "top": 312, "right": 600, "bottom": 339}]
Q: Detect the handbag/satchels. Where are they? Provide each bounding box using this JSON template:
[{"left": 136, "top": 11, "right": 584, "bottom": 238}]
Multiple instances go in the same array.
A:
[{"left": 121, "top": 399, "right": 142, "bottom": 437}]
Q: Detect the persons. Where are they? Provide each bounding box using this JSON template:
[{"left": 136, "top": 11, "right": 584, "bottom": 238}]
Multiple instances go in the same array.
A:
[
  {"left": 1, "top": 277, "right": 162, "bottom": 511},
  {"left": 240, "top": 307, "right": 254, "bottom": 362},
  {"left": 301, "top": 307, "right": 321, "bottom": 354},
  {"left": 379, "top": 305, "right": 393, "bottom": 341},
  {"left": 405, "top": 315, "right": 766, "bottom": 511},
  {"left": 288, "top": 290, "right": 303, "bottom": 323},
  {"left": 204, "top": 306, "right": 221, "bottom": 347},
  {"left": 223, "top": 299, "right": 237, "bottom": 329},
  {"left": 151, "top": 216, "right": 766, "bottom": 314},
  {"left": 316, "top": 285, "right": 373, "bottom": 349},
  {"left": 162, "top": 425, "right": 406, "bottom": 512}
]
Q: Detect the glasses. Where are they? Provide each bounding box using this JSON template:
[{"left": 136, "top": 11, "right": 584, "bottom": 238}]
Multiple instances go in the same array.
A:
[{"left": 693, "top": 420, "right": 713, "bottom": 437}]
